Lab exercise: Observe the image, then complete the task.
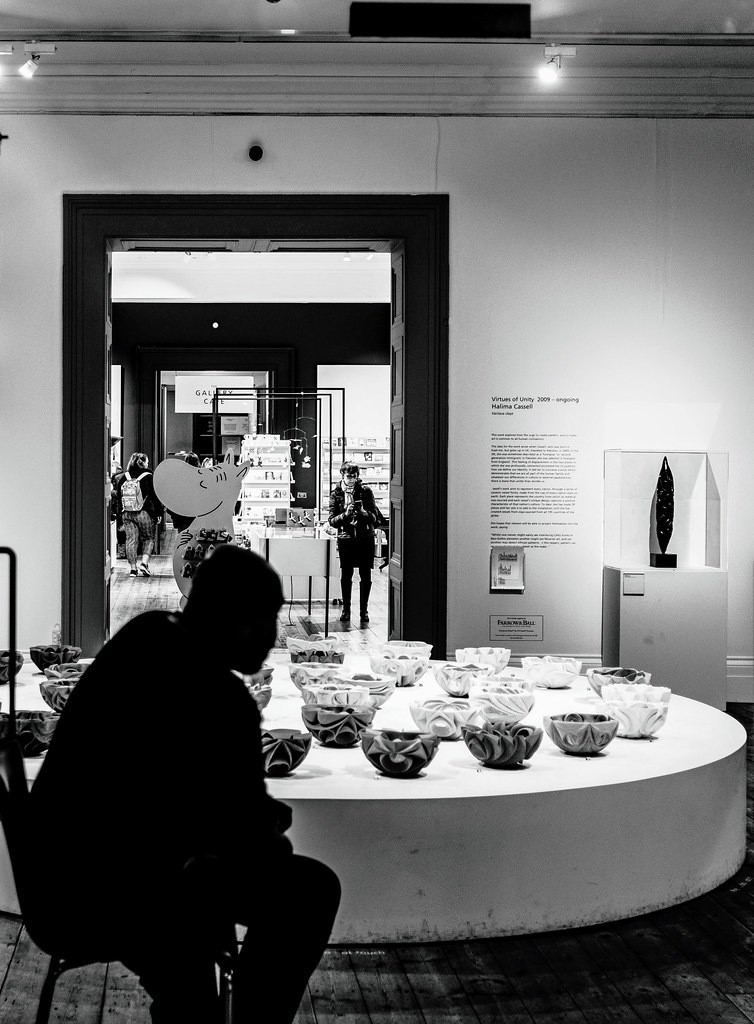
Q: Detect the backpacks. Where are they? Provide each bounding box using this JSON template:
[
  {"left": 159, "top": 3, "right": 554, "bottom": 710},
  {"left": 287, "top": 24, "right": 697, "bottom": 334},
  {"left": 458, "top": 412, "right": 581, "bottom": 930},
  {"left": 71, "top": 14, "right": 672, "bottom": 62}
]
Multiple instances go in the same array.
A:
[{"left": 121, "top": 471, "right": 153, "bottom": 511}]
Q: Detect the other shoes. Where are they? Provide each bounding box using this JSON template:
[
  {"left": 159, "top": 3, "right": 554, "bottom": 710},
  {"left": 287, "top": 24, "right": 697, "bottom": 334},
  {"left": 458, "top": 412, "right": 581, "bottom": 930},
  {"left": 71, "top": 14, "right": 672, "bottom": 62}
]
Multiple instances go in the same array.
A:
[
  {"left": 139, "top": 563, "right": 150, "bottom": 577},
  {"left": 130, "top": 570, "right": 138, "bottom": 576}
]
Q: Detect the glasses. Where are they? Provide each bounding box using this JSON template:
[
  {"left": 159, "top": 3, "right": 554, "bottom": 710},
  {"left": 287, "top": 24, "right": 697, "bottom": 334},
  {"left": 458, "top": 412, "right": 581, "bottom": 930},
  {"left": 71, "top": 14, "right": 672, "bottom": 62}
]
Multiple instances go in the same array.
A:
[{"left": 346, "top": 474, "right": 357, "bottom": 479}]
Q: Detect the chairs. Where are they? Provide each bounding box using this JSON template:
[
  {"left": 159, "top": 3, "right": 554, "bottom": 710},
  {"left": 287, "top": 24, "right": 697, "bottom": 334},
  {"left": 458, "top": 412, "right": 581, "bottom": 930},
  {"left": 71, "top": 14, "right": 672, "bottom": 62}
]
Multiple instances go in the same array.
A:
[{"left": 0, "top": 714, "right": 233, "bottom": 1024}]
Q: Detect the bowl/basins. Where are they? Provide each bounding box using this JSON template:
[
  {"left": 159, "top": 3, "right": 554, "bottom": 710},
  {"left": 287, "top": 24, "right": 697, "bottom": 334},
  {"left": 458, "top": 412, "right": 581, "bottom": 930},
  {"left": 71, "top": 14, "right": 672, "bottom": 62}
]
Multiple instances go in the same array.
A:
[
  {"left": 236, "top": 662, "right": 274, "bottom": 712},
  {"left": 286, "top": 632, "right": 433, "bottom": 747},
  {"left": 463, "top": 722, "right": 544, "bottom": 770},
  {"left": 0, "top": 710, "right": 60, "bottom": 758},
  {"left": 0, "top": 649, "right": 24, "bottom": 687},
  {"left": 521, "top": 653, "right": 581, "bottom": 690},
  {"left": 439, "top": 647, "right": 535, "bottom": 723},
  {"left": 29, "top": 644, "right": 92, "bottom": 713},
  {"left": 408, "top": 696, "right": 484, "bottom": 742},
  {"left": 260, "top": 726, "right": 313, "bottom": 778},
  {"left": 357, "top": 726, "right": 441, "bottom": 779},
  {"left": 543, "top": 711, "right": 620, "bottom": 760},
  {"left": 586, "top": 665, "right": 673, "bottom": 738}
]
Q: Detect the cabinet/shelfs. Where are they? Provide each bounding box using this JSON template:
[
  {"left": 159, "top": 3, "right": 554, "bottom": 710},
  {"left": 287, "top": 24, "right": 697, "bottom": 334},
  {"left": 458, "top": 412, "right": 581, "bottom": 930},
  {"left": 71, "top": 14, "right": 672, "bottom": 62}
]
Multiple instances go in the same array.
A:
[{"left": 236, "top": 434, "right": 343, "bottom": 599}]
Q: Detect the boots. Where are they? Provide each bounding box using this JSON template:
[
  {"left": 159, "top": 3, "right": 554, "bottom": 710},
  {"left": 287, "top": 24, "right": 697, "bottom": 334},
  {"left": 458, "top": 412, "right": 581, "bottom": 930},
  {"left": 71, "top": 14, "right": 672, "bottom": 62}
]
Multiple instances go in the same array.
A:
[
  {"left": 360, "top": 581, "right": 373, "bottom": 622},
  {"left": 116, "top": 544, "right": 125, "bottom": 559},
  {"left": 339, "top": 580, "right": 353, "bottom": 622}
]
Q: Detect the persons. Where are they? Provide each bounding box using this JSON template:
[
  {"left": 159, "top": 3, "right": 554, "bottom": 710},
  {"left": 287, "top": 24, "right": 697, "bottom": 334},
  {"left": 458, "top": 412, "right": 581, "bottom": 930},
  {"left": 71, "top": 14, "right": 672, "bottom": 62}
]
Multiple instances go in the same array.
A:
[
  {"left": 166, "top": 451, "right": 203, "bottom": 534},
  {"left": 113, "top": 452, "right": 163, "bottom": 577},
  {"left": 328, "top": 461, "right": 378, "bottom": 623},
  {"left": 17, "top": 542, "right": 344, "bottom": 1024}
]
